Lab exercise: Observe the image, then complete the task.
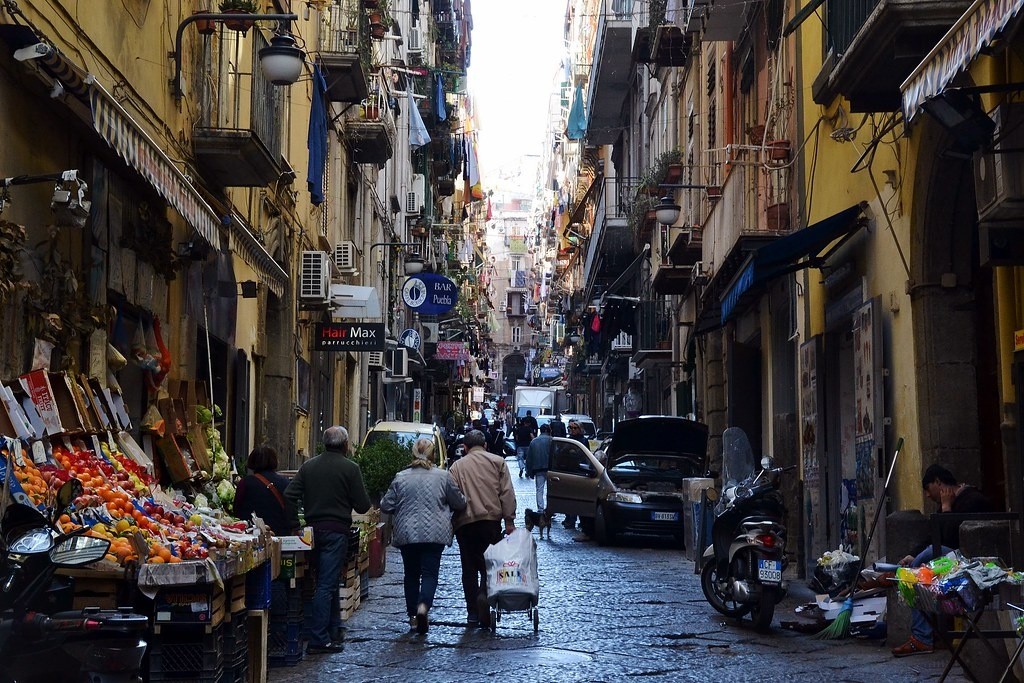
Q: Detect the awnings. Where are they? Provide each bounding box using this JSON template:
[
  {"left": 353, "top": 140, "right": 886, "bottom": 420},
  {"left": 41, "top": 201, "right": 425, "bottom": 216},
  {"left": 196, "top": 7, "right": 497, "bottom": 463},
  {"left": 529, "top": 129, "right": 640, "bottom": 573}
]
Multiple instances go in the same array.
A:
[
  {"left": 194, "top": 181, "right": 289, "bottom": 299},
  {"left": 719, "top": 201, "right": 871, "bottom": 318},
  {"left": 899, "top": 0, "right": 1024, "bottom": 123},
  {"left": 0, "top": 19, "right": 220, "bottom": 248},
  {"left": 600, "top": 248, "right": 646, "bottom": 303}
]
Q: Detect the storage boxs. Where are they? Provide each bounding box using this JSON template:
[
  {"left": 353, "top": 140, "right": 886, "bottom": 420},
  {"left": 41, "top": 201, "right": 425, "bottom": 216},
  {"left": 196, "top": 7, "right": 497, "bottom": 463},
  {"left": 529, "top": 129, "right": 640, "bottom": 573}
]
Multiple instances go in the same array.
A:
[{"left": 0, "top": 366, "right": 385, "bottom": 683}]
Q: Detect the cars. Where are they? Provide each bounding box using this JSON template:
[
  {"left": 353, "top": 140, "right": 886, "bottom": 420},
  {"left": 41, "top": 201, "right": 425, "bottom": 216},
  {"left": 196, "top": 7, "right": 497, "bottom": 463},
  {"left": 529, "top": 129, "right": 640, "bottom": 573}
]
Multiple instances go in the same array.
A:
[
  {"left": 533, "top": 413, "right": 598, "bottom": 446},
  {"left": 359, "top": 418, "right": 451, "bottom": 474},
  {"left": 545, "top": 415, "right": 718, "bottom": 546}
]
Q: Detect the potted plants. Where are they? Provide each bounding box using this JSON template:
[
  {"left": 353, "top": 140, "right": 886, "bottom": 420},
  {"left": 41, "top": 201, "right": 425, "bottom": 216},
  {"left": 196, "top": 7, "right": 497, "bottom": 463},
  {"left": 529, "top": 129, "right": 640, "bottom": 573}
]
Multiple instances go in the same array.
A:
[
  {"left": 653, "top": 183, "right": 723, "bottom": 225},
  {"left": 361, "top": 0, "right": 395, "bottom": 38},
  {"left": 744, "top": 119, "right": 767, "bottom": 144},
  {"left": 362, "top": 96, "right": 379, "bottom": 118},
  {"left": 218, "top": 0, "right": 261, "bottom": 30},
  {"left": 627, "top": 144, "right": 683, "bottom": 220},
  {"left": 708, "top": 182, "right": 719, "bottom": 196},
  {"left": 411, "top": 219, "right": 433, "bottom": 237},
  {"left": 767, "top": 136, "right": 792, "bottom": 159}
]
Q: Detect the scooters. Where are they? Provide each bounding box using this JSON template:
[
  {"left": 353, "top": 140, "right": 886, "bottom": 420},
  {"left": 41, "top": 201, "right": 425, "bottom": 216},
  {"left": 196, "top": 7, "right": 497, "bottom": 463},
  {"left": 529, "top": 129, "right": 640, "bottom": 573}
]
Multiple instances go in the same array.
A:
[
  {"left": 0, "top": 477, "right": 150, "bottom": 683},
  {"left": 698, "top": 426, "right": 800, "bottom": 633}
]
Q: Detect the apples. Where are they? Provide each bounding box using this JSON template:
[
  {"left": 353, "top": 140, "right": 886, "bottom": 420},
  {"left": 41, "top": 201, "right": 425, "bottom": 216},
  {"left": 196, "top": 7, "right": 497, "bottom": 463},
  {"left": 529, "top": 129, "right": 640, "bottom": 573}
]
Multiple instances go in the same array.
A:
[{"left": 85, "top": 442, "right": 226, "bottom": 551}]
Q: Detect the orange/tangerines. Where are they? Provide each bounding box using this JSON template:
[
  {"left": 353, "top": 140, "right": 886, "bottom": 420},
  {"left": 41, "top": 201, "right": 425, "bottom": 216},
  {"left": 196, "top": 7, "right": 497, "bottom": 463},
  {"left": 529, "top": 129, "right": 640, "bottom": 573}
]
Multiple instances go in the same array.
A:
[
  {"left": 2, "top": 448, "right": 129, "bottom": 509},
  {"left": 59, "top": 502, "right": 209, "bottom": 564}
]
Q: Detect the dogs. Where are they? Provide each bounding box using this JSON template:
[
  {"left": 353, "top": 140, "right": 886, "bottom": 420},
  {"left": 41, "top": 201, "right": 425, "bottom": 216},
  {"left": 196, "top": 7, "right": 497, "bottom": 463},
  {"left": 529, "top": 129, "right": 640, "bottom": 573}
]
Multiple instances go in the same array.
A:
[{"left": 525, "top": 508, "right": 557, "bottom": 541}]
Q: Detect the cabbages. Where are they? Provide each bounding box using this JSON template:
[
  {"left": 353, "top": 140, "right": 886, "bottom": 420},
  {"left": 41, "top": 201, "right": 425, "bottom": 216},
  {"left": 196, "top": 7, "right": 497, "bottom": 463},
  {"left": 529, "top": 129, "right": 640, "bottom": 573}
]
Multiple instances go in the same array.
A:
[{"left": 195, "top": 403, "right": 235, "bottom": 505}]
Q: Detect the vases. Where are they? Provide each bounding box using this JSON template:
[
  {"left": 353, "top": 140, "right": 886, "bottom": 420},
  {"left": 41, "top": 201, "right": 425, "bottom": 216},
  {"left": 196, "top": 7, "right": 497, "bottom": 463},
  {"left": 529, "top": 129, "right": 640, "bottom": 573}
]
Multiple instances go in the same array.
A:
[{"left": 193, "top": 10, "right": 216, "bottom": 35}]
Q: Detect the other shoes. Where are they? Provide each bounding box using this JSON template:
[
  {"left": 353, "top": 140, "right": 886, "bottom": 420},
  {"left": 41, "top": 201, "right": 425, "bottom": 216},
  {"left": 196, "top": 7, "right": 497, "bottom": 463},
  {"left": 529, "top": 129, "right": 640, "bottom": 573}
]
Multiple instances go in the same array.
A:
[
  {"left": 409, "top": 615, "right": 417, "bottom": 628},
  {"left": 562, "top": 520, "right": 575, "bottom": 528},
  {"left": 876, "top": 572, "right": 895, "bottom": 586},
  {"left": 336, "top": 630, "right": 348, "bottom": 643},
  {"left": 892, "top": 635, "right": 934, "bottom": 656},
  {"left": 306, "top": 643, "right": 345, "bottom": 654},
  {"left": 417, "top": 603, "right": 430, "bottom": 633},
  {"left": 468, "top": 614, "right": 478, "bottom": 624},
  {"left": 478, "top": 597, "right": 490, "bottom": 628}
]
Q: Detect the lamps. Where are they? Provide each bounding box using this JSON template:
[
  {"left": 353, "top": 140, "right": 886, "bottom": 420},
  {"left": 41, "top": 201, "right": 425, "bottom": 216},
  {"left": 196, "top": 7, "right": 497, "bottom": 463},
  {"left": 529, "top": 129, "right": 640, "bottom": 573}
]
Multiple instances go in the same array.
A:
[
  {"left": 570, "top": 323, "right": 582, "bottom": 342},
  {"left": 371, "top": 241, "right": 423, "bottom": 273},
  {"left": 237, "top": 280, "right": 257, "bottom": 299},
  {"left": 49, "top": 189, "right": 93, "bottom": 230},
  {"left": 171, "top": 13, "right": 305, "bottom": 101}
]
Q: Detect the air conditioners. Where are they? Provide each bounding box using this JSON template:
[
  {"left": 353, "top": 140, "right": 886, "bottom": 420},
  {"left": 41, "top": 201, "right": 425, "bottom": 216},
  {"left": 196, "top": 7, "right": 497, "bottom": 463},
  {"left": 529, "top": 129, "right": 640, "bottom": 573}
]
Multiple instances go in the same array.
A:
[
  {"left": 499, "top": 306, "right": 506, "bottom": 312},
  {"left": 409, "top": 28, "right": 423, "bottom": 50},
  {"left": 406, "top": 192, "right": 420, "bottom": 213},
  {"left": 335, "top": 240, "right": 363, "bottom": 268},
  {"left": 299, "top": 250, "right": 333, "bottom": 299},
  {"left": 500, "top": 301, "right": 506, "bottom": 305}
]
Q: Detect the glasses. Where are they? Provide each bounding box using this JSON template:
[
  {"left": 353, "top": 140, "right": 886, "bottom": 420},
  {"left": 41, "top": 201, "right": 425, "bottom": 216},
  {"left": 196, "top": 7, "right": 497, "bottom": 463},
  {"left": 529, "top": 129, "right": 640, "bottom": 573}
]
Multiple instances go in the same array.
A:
[{"left": 571, "top": 426, "right": 578, "bottom": 430}]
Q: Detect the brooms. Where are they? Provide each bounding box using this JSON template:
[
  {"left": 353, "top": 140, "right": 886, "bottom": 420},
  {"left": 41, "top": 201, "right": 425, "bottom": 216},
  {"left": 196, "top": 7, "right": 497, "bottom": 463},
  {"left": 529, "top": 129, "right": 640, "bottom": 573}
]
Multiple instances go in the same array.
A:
[{"left": 806, "top": 437, "right": 904, "bottom": 640}]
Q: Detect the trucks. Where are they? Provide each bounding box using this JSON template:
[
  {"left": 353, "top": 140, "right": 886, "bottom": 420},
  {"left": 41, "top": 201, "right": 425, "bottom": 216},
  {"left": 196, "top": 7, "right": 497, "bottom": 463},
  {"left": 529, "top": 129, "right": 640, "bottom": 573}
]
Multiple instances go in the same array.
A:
[{"left": 514, "top": 386, "right": 555, "bottom": 423}]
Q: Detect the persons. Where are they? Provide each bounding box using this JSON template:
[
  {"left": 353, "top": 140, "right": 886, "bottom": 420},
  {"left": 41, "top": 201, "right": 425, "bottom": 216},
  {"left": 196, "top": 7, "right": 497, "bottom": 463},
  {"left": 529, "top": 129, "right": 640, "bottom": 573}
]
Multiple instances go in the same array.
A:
[
  {"left": 515, "top": 422, "right": 536, "bottom": 477},
  {"left": 550, "top": 414, "right": 566, "bottom": 438},
  {"left": 379, "top": 439, "right": 465, "bottom": 634},
  {"left": 877, "top": 464, "right": 990, "bottom": 656},
  {"left": 282, "top": 425, "right": 370, "bottom": 653},
  {"left": 235, "top": 446, "right": 302, "bottom": 533},
  {"left": 522, "top": 410, "right": 538, "bottom": 436},
  {"left": 447, "top": 430, "right": 516, "bottom": 628},
  {"left": 527, "top": 424, "right": 556, "bottom": 513},
  {"left": 471, "top": 398, "right": 513, "bottom": 457},
  {"left": 561, "top": 418, "right": 607, "bottom": 541}
]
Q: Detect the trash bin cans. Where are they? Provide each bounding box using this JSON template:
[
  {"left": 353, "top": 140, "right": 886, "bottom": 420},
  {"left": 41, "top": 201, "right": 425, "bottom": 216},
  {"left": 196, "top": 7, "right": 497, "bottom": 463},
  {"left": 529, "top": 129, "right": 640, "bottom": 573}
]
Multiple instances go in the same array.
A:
[{"left": 682, "top": 478, "right": 714, "bottom": 562}]
